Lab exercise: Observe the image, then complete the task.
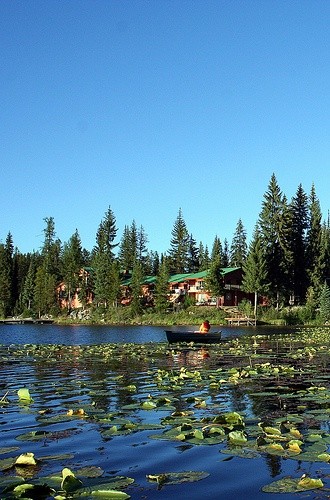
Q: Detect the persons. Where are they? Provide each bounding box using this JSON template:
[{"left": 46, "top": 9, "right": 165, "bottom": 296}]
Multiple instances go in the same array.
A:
[{"left": 200, "top": 320, "right": 211, "bottom": 333}]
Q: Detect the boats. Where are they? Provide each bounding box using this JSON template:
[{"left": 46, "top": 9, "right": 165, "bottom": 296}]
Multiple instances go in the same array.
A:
[{"left": 165, "top": 330, "right": 221, "bottom": 343}]
